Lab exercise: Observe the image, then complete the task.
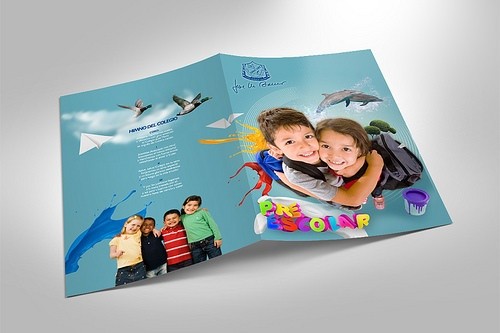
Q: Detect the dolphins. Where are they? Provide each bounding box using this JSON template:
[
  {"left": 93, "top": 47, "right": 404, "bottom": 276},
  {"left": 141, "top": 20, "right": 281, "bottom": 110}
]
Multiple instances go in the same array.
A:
[
  {"left": 349, "top": 93, "right": 383, "bottom": 106},
  {"left": 316, "top": 90, "right": 364, "bottom": 113}
]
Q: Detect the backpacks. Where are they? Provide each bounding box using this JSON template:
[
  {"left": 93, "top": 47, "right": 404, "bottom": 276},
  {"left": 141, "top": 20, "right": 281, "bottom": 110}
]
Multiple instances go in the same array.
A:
[
  {"left": 370, "top": 133, "right": 422, "bottom": 191},
  {"left": 256, "top": 149, "right": 326, "bottom": 197}
]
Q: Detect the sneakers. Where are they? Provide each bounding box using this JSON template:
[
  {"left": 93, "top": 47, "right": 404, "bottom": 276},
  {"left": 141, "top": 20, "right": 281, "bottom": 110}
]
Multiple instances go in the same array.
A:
[
  {"left": 341, "top": 204, "right": 361, "bottom": 211},
  {"left": 372, "top": 197, "right": 384, "bottom": 209}
]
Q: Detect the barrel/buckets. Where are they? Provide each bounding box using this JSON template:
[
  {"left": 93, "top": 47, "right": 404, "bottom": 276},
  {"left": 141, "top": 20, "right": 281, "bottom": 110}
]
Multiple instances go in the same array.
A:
[{"left": 403, "top": 188, "right": 429, "bottom": 216}]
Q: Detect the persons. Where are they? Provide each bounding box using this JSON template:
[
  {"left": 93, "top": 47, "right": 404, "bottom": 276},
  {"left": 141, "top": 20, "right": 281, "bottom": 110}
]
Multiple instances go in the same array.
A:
[
  {"left": 158, "top": 209, "right": 192, "bottom": 273},
  {"left": 316, "top": 119, "right": 389, "bottom": 209},
  {"left": 259, "top": 106, "right": 386, "bottom": 205},
  {"left": 140, "top": 216, "right": 167, "bottom": 277},
  {"left": 178, "top": 195, "right": 223, "bottom": 265},
  {"left": 109, "top": 214, "right": 163, "bottom": 288}
]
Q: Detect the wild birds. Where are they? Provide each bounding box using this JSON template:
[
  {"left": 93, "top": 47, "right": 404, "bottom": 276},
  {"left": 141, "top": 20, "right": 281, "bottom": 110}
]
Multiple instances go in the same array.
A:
[
  {"left": 173, "top": 93, "right": 213, "bottom": 116},
  {"left": 118, "top": 99, "right": 152, "bottom": 119}
]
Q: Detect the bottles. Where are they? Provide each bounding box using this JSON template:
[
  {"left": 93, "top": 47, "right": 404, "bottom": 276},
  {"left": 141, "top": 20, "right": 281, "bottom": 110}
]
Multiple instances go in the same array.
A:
[{"left": 374, "top": 191, "right": 385, "bottom": 210}]
